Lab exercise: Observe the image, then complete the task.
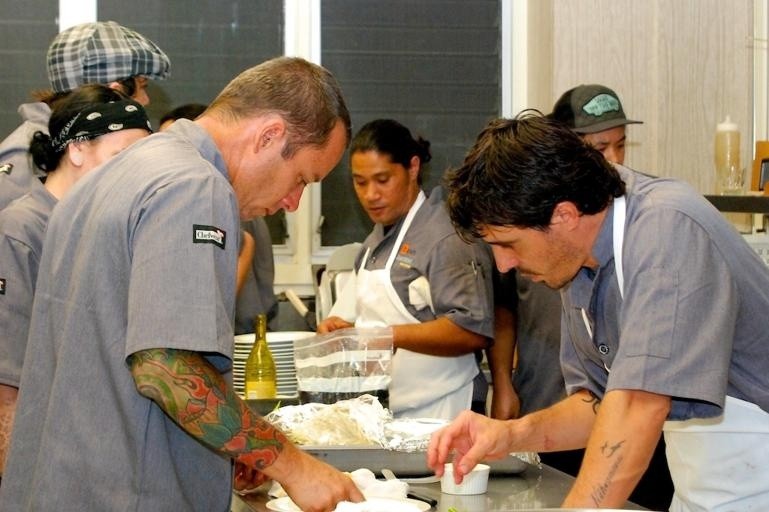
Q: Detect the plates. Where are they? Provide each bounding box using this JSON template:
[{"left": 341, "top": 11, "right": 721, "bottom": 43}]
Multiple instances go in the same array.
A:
[
  {"left": 233, "top": 330, "right": 318, "bottom": 399},
  {"left": 264, "top": 493, "right": 432, "bottom": 512}
]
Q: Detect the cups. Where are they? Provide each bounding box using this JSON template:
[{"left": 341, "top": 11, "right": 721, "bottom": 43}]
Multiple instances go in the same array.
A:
[{"left": 721, "top": 168, "right": 744, "bottom": 193}]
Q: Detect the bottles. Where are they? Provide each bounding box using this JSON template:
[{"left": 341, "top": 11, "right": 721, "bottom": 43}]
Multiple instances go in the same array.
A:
[
  {"left": 714, "top": 114, "right": 741, "bottom": 194},
  {"left": 233, "top": 312, "right": 279, "bottom": 496}
]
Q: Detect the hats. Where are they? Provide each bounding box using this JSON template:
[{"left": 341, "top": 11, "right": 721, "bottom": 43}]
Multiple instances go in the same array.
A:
[
  {"left": 47, "top": 18, "right": 171, "bottom": 93},
  {"left": 553, "top": 82, "right": 644, "bottom": 133}
]
